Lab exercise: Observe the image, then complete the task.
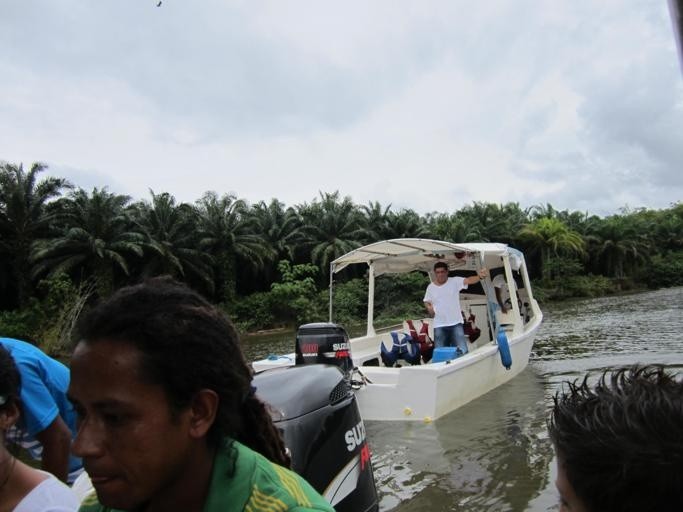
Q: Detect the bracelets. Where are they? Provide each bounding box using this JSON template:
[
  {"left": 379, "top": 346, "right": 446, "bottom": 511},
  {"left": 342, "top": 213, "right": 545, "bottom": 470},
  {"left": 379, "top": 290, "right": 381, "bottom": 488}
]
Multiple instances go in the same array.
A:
[{"left": 479, "top": 276, "right": 482, "bottom": 280}]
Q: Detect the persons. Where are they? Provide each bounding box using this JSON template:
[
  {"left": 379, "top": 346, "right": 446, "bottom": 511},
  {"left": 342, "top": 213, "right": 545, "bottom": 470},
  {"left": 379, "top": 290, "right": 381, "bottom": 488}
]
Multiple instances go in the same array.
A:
[
  {"left": 66, "top": 278, "right": 334, "bottom": 512},
  {"left": 0, "top": 341, "right": 71, "bottom": 512},
  {"left": 486, "top": 272, "right": 523, "bottom": 342},
  {"left": 422, "top": 262, "right": 488, "bottom": 354},
  {"left": 1, "top": 334, "right": 83, "bottom": 485},
  {"left": 547, "top": 362, "right": 681, "bottom": 509}
]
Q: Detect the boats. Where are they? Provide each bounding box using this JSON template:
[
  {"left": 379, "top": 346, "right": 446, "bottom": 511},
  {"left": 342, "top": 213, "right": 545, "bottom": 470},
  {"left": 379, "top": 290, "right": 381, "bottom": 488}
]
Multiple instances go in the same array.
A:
[
  {"left": 249, "top": 234, "right": 547, "bottom": 425},
  {"left": 348, "top": 366, "right": 547, "bottom": 476}
]
